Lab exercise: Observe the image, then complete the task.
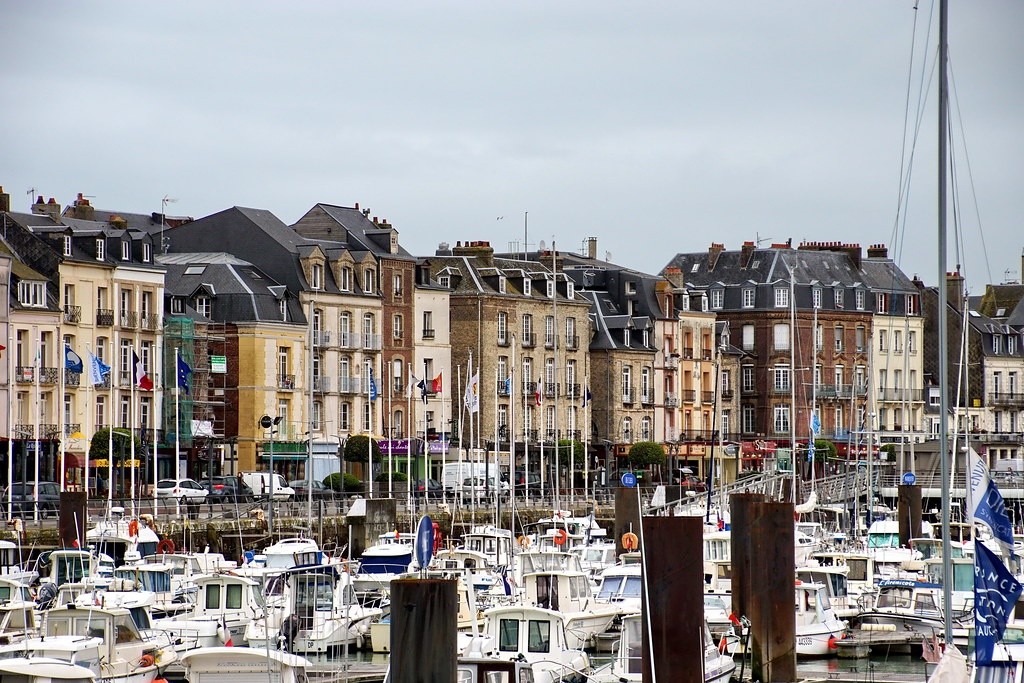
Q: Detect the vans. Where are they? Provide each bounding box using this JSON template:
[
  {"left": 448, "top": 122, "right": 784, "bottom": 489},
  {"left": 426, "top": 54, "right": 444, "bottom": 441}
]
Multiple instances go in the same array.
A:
[{"left": 242, "top": 471, "right": 296, "bottom": 502}]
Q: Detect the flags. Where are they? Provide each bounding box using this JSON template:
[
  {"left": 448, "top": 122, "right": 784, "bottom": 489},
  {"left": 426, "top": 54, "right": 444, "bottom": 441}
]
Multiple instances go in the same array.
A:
[
  {"left": 370, "top": 368, "right": 377, "bottom": 400},
  {"left": 535, "top": 377, "right": 541, "bottom": 406},
  {"left": 810, "top": 410, "right": 820, "bottom": 435},
  {"left": 965, "top": 447, "right": 1015, "bottom": 560},
  {"left": 405, "top": 373, "right": 420, "bottom": 399},
  {"left": 64, "top": 344, "right": 83, "bottom": 373},
  {"left": 971, "top": 537, "right": 1023, "bottom": 666},
  {"left": 418, "top": 379, "right": 428, "bottom": 404},
  {"left": 432, "top": 373, "right": 442, "bottom": 393},
  {"left": 464, "top": 374, "right": 478, "bottom": 413},
  {"left": 582, "top": 386, "right": 592, "bottom": 407},
  {"left": 133, "top": 349, "right": 153, "bottom": 391},
  {"left": 178, "top": 353, "right": 192, "bottom": 395},
  {"left": 88, "top": 350, "right": 110, "bottom": 385}
]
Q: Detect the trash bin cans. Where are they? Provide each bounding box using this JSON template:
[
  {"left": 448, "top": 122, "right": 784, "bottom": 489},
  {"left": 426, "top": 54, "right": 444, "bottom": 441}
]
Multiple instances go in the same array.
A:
[
  {"left": 595, "top": 490, "right": 603, "bottom": 505},
  {"left": 187, "top": 502, "right": 200, "bottom": 520}
]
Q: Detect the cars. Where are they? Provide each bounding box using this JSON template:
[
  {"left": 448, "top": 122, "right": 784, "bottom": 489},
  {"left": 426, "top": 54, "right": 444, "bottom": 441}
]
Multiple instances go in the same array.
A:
[
  {"left": 287, "top": 479, "right": 336, "bottom": 502},
  {"left": 151, "top": 478, "right": 210, "bottom": 505}
]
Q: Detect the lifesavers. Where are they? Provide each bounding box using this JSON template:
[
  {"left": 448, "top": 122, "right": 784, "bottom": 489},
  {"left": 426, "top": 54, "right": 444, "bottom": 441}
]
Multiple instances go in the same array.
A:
[
  {"left": 129, "top": 521, "right": 137, "bottom": 537},
  {"left": 517, "top": 536, "right": 529, "bottom": 546},
  {"left": 795, "top": 580, "right": 803, "bottom": 585},
  {"left": 157, "top": 539, "right": 175, "bottom": 554},
  {"left": 95, "top": 590, "right": 107, "bottom": 606},
  {"left": 554, "top": 529, "right": 566, "bottom": 545},
  {"left": 621, "top": 532, "right": 638, "bottom": 549}
]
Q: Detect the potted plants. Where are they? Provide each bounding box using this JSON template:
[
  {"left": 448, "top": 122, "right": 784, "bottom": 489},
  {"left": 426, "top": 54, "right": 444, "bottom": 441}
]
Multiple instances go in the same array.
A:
[{"left": 344, "top": 434, "right": 408, "bottom": 503}]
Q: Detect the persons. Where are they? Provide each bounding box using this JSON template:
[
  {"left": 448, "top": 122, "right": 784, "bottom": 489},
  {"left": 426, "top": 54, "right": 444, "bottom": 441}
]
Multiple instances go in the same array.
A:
[{"left": 1006, "top": 467, "right": 1013, "bottom": 476}]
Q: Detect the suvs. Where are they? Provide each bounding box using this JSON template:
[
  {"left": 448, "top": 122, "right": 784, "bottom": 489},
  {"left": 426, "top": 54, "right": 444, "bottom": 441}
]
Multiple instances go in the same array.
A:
[
  {"left": 0, "top": 481, "right": 69, "bottom": 520},
  {"left": 198, "top": 475, "right": 254, "bottom": 505}
]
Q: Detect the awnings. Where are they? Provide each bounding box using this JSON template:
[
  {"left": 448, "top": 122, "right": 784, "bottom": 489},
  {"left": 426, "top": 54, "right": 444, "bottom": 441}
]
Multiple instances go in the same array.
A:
[{"left": 65, "top": 452, "right": 140, "bottom": 468}]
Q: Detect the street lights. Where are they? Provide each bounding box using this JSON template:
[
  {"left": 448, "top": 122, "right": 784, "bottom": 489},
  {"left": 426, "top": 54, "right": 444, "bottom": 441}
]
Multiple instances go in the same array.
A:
[{"left": 260, "top": 416, "right": 284, "bottom": 536}]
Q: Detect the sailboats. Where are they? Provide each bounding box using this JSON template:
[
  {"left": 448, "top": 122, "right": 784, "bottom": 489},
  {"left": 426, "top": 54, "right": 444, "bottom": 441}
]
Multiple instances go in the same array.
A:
[{"left": 0, "top": 0, "right": 1024, "bottom": 683}]
